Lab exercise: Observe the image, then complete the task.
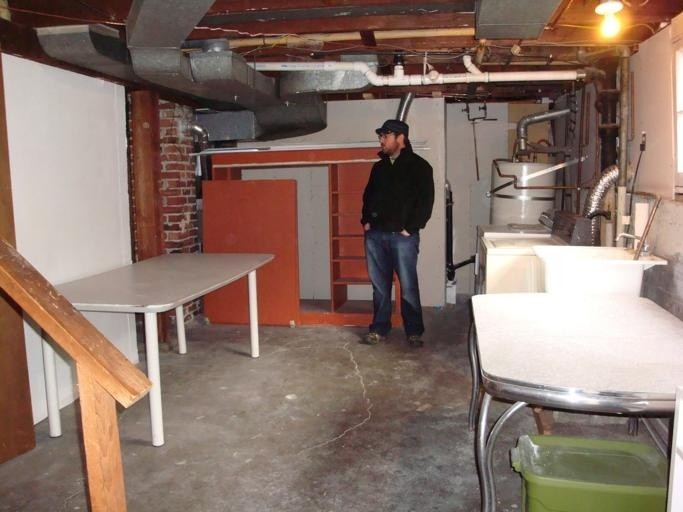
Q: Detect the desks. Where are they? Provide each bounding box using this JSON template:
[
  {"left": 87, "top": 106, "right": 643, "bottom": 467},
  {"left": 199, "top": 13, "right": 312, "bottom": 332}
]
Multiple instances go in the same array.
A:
[
  {"left": 35, "top": 250, "right": 277, "bottom": 449},
  {"left": 465, "top": 290, "right": 681, "bottom": 512}
]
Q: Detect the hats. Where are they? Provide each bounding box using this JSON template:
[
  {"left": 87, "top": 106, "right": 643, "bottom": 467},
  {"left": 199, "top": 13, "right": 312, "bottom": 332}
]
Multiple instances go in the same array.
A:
[{"left": 376, "top": 120, "right": 408, "bottom": 134}]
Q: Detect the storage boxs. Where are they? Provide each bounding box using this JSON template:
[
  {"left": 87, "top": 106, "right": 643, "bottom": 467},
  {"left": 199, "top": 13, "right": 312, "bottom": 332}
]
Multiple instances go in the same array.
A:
[{"left": 509, "top": 433, "right": 668, "bottom": 511}]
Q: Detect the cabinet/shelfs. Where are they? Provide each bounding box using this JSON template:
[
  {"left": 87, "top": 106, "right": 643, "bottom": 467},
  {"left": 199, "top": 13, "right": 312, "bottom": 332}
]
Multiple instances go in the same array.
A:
[
  {"left": 328, "top": 182, "right": 398, "bottom": 287},
  {"left": 473, "top": 223, "right": 552, "bottom": 295}
]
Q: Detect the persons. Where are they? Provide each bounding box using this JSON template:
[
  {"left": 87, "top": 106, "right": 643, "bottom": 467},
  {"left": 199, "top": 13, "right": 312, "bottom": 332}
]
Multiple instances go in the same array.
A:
[{"left": 360, "top": 119, "right": 434, "bottom": 348}]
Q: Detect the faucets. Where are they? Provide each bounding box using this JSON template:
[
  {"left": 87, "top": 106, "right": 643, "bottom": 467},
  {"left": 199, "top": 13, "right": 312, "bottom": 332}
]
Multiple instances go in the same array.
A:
[{"left": 613, "top": 233, "right": 652, "bottom": 257}]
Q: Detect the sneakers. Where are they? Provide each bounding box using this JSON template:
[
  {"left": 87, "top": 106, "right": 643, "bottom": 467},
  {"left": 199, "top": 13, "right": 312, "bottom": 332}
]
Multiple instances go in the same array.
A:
[
  {"left": 407, "top": 335, "right": 423, "bottom": 346},
  {"left": 364, "top": 333, "right": 386, "bottom": 344}
]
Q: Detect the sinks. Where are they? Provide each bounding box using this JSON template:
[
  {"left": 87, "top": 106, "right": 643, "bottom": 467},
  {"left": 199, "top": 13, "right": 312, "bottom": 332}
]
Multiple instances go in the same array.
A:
[{"left": 531, "top": 242, "right": 669, "bottom": 296}]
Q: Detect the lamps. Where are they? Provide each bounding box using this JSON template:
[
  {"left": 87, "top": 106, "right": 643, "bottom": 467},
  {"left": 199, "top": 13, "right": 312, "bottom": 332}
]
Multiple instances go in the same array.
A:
[{"left": 591, "top": 2, "right": 656, "bottom": 42}]
